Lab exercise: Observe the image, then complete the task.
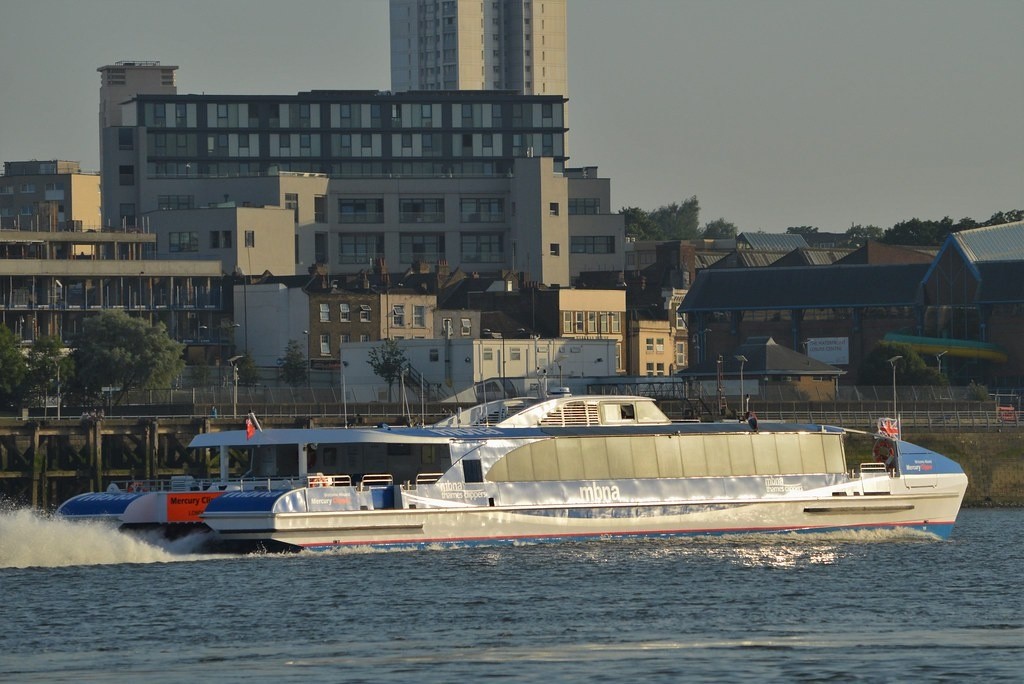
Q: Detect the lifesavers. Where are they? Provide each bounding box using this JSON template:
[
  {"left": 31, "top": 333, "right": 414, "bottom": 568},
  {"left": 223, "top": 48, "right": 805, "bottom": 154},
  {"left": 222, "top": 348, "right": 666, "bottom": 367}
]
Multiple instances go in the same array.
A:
[
  {"left": 872, "top": 439, "right": 894, "bottom": 465},
  {"left": 128, "top": 482, "right": 149, "bottom": 493}
]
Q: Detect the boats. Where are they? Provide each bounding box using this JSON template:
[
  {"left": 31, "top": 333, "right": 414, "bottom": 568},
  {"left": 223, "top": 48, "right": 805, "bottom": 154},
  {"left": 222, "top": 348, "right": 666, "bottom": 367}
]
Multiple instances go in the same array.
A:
[{"left": 54, "top": 385, "right": 971, "bottom": 556}]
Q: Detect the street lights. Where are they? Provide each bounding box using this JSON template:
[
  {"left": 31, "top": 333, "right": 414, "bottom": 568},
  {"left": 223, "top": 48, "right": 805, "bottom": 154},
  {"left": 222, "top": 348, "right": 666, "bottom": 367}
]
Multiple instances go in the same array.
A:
[
  {"left": 401, "top": 368, "right": 409, "bottom": 417},
  {"left": 937, "top": 350, "right": 948, "bottom": 374},
  {"left": 481, "top": 328, "right": 526, "bottom": 398},
  {"left": 216, "top": 324, "right": 242, "bottom": 402},
  {"left": 887, "top": 355, "right": 903, "bottom": 420},
  {"left": 227, "top": 355, "right": 243, "bottom": 404},
  {"left": 734, "top": 355, "right": 748, "bottom": 416},
  {"left": 553, "top": 354, "right": 569, "bottom": 387},
  {"left": 529, "top": 334, "right": 541, "bottom": 373},
  {"left": 302, "top": 331, "right": 311, "bottom": 387}
]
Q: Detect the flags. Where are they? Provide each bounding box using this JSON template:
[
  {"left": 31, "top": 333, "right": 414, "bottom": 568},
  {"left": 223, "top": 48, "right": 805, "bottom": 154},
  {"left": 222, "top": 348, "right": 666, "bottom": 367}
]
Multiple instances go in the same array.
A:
[
  {"left": 877, "top": 417, "right": 899, "bottom": 441},
  {"left": 246, "top": 413, "right": 255, "bottom": 440}
]
